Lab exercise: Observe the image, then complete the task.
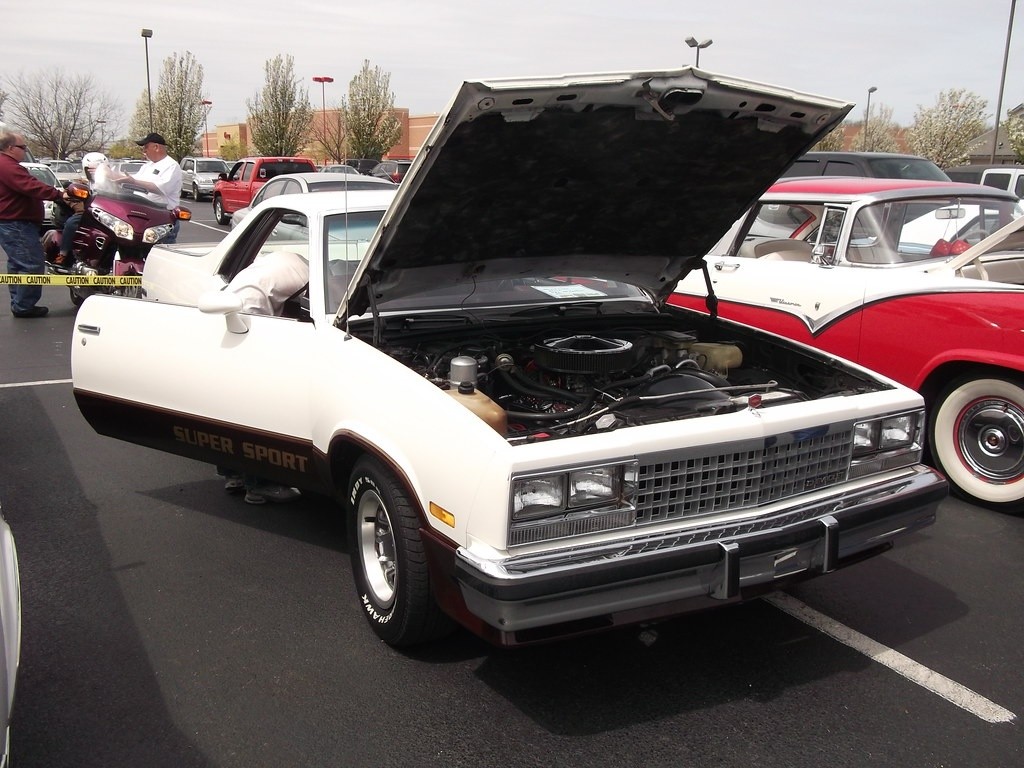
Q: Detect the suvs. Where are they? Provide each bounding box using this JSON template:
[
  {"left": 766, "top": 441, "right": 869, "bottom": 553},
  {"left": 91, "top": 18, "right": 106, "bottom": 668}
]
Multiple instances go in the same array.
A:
[
  {"left": 211, "top": 157, "right": 317, "bottom": 225},
  {"left": 180, "top": 156, "right": 232, "bottom": 202}
]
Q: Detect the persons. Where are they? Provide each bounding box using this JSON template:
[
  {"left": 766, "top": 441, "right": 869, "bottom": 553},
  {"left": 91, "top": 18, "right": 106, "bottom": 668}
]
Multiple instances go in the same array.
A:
[
  {"left": 89, "top": 132, "right": 183, "bottom": 244},
  {"left": 0, "top": 125, "right": 70, "bottom": 318},
  {"left": 217, "top": 252, "right": 309, "bottom": 505}
]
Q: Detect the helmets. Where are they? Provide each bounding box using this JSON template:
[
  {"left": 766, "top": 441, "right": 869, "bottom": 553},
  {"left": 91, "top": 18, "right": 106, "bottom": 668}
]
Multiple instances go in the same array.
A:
[{"left": 83, "top": 152, "right": 110, "bottom": 184}]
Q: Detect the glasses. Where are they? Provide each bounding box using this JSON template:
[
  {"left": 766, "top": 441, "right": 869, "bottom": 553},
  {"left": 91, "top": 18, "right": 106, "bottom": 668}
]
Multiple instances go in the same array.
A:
[{"left": 7, "top": 145, "right": 27, "bottom": 150}]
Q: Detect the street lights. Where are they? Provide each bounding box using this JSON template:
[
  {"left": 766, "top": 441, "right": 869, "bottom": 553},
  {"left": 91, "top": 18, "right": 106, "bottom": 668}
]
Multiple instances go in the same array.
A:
[
  {"left": 199, "top": 101, "right": 212, "bottom": 156},
  {"left": 861, "top": 87, "right": 877, "bottom": 151},
  {"left": 685, "top": 36, "right": 713, "bottom": 67},
  {"left": 141, "top": 29, "right": 153, "bottom": 134},
  {"left": 313, "top": 76, "right": 334, "bottom": 166},
  {"left": 98, "top": 120, "right": 106, "bottom": 151}
]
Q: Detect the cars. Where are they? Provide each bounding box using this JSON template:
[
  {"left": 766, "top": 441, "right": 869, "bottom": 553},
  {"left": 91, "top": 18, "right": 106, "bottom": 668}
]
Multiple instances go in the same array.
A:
[
  {"left": 17, "top": 145, "right": 90, "bottom": 235},
  {"left": 71, "top": 67, "right": 1024, "bottom": 653},
  {"left": 0, "top": 515, "right": 23, "bottom": 765},
  {"left": 109, "top": 158, "right": 147, "bottom": 175}
]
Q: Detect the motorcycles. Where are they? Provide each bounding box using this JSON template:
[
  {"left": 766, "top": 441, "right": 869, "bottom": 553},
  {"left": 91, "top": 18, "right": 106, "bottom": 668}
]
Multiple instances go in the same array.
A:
[{"left": 44, "top": 159, "right": 192, "bottom": 308}]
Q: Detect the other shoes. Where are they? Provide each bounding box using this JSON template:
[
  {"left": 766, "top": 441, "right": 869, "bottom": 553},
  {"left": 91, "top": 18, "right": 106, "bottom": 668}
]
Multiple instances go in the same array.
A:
[
  {"left": 55, "top": 253, "right": 67, "bottom": 264},
  {"left": 13, "top": 306, "right": 48, "bottom": 317}
]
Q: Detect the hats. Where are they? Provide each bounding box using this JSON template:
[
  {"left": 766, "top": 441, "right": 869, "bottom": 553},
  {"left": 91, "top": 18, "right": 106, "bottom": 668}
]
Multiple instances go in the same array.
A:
[{"left": 134, "top": 133, "right": 165, "bottom": 146}]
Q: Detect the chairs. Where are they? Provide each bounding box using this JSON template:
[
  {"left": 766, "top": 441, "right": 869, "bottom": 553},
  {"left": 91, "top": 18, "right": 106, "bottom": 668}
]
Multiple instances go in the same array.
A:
[{"left": 740, "top": 237, "right": 903, "bottom": 264}]
